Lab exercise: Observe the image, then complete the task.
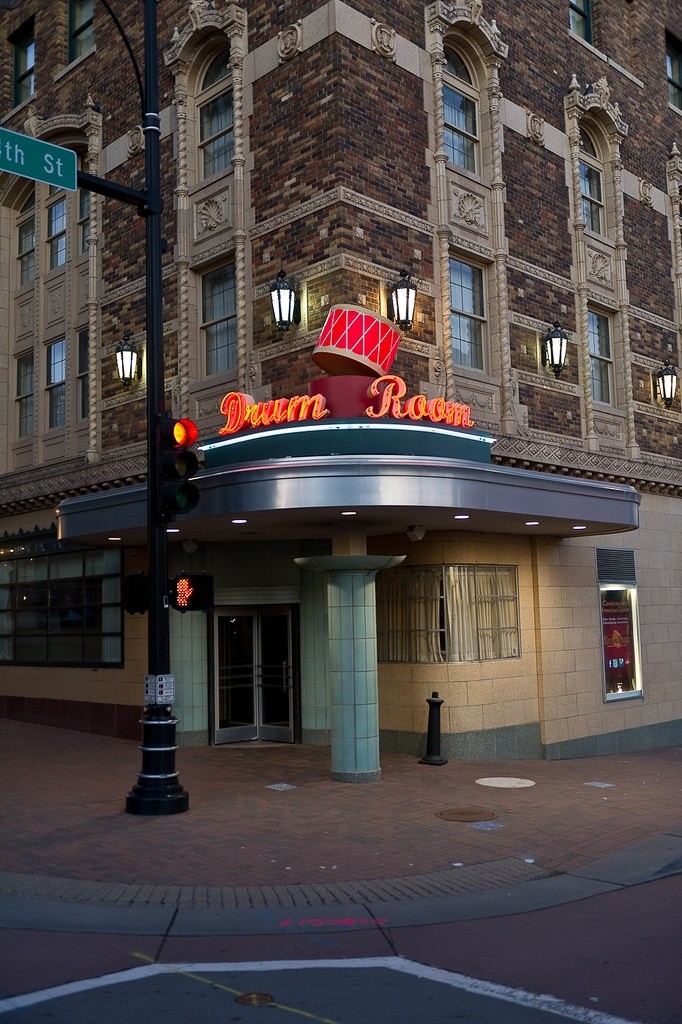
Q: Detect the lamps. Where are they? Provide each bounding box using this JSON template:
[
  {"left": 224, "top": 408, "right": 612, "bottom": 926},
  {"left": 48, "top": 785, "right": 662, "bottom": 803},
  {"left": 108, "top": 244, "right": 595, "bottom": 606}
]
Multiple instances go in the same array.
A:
[
  {"left": 538, "top": 321, "right": 569, "bottom": 379},
  {"left": 270, "top": 267, "right": 303, "bottom": 331},
  {"left": 407, "top": 524, "right": 426, "bottom": 543},
  {"left": 183, "top": 538, "right": 200, "bottom": 554},
  {"left": 388, "top": 270, "right": 417, "bottom": 332},
  {"left": 652, "top": 358, "right": 677, "bottom": 408},
  {"left": 117, "top": 330, "right": 143, "bottom": 388}
]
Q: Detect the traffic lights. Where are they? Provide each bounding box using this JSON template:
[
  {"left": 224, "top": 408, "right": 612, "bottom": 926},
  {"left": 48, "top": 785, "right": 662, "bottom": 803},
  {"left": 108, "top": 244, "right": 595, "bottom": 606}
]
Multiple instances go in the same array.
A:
[
  {"left": 123, "top": 574, "right": 150, "bottom": 615},
  {"left": 155, "top": 415, "right": 200, "bottom": 524},
  {"left": 172, "top": 573, "right": 215, "bottom": 612}
]
